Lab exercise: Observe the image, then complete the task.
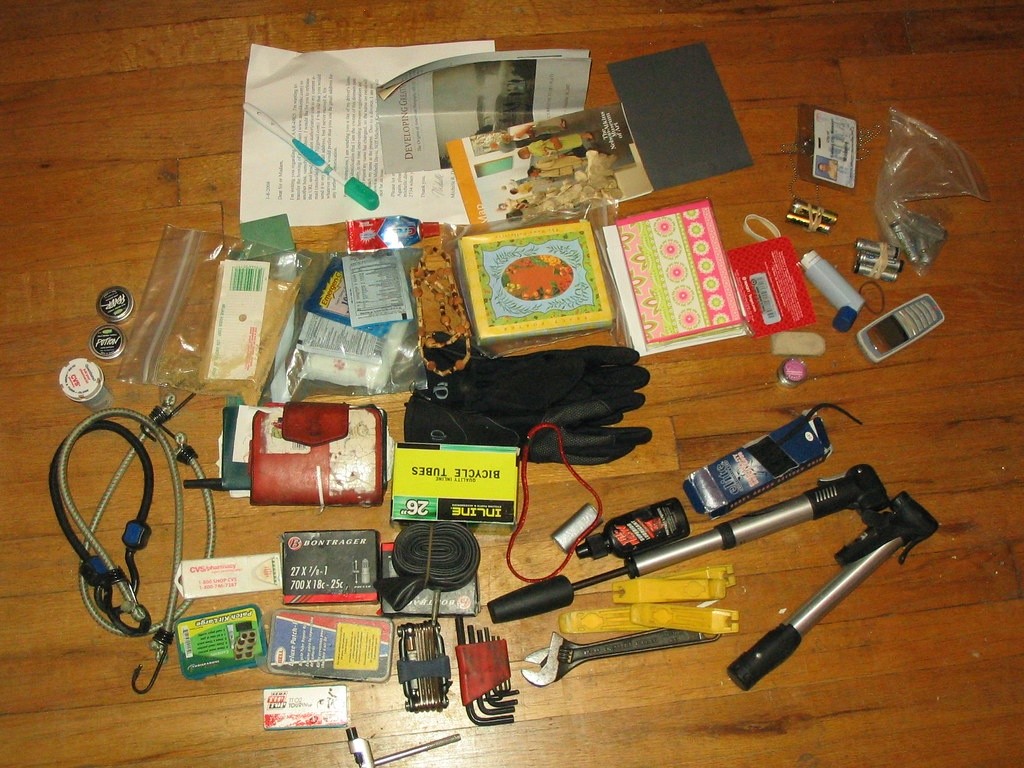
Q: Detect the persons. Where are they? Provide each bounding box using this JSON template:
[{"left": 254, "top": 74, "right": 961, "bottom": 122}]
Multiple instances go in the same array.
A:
[
  {"left": 817, "top": 162, "right": 837, "bottom": 179},
  {"left": 498, "top": 118, "right": 593, "bottom": 221}
]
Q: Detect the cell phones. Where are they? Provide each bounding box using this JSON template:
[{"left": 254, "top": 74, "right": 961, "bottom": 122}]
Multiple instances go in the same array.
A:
[{"left": 856, "top": 294, "right": 945, "bottom": 364}]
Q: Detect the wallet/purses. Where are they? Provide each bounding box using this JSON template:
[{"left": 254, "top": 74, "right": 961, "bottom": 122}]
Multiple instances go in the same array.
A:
[{"left": 250, "top": 401, "right": 390, "bottom": 509}]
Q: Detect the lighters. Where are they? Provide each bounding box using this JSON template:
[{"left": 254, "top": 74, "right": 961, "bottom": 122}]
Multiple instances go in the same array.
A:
[{"left": 797, "top": 251, "right": 867, "bottom": 313}]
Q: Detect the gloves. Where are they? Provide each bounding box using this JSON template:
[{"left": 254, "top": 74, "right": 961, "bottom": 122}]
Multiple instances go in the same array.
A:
[
  {"left": 404, "top": 389, "right": 652, "bottom": 465},
  {"left": 423, "top": 331, "right": 650, "bottom": 416}
]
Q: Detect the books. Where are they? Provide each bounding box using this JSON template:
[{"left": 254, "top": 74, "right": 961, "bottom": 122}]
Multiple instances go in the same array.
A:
[{"left": 459, "top": 221, "right": 612, "bottom": 344}]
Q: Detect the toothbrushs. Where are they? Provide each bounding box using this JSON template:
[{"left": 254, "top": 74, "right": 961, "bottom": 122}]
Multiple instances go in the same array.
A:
[{"left": 243, "top": 100, "right": 379, "bottom": 210}]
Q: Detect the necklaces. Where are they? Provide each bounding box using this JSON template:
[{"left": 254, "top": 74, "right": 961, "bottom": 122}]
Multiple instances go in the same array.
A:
[{"left": 410, "top": 246, "right": 473, "bottom": 375}]
